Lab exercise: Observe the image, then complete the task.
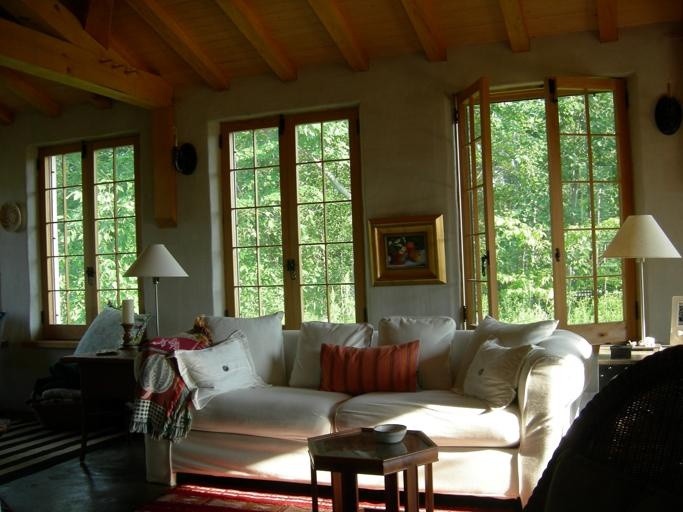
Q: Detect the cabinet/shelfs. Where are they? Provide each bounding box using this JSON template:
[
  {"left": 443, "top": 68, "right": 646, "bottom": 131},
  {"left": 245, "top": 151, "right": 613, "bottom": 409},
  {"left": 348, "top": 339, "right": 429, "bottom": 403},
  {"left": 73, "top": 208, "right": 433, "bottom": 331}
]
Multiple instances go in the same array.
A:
[
  {"left": 597, "top": 341, "right": 662, "bottom": 390},
  {"left": 63, "top": 347, "right": 142, "bottom": 467}
]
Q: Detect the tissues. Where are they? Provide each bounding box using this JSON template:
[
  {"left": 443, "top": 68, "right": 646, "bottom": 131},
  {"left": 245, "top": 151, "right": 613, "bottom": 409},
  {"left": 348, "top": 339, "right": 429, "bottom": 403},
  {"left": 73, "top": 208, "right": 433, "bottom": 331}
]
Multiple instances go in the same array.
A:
[{"left": 629, "top": 337, "right": 663, "bottom": 361}]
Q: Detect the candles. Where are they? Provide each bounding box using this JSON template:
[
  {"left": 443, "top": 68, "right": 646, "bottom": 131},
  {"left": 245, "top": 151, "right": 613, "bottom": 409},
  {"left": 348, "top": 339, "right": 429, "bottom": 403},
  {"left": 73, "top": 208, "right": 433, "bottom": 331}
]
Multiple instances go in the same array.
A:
[{"left": 123, "top": 300, "right": 136, "bottom": 324}]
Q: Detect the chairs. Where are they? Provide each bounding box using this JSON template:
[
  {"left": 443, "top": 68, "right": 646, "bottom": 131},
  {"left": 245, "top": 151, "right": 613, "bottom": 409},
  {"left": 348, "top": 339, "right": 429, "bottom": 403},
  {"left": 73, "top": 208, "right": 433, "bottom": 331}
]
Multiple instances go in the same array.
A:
[
  {"left": 524, "top": 345, "right": 683, "bottom": 511},
  {"left": 25, "top": 323, "right": 148, "bottom": 429}
]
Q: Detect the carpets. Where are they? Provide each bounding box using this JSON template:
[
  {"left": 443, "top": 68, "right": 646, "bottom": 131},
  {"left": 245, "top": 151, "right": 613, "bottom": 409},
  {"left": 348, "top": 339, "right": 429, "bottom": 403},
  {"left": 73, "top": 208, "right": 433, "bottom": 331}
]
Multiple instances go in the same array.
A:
[
  {"left": 137, "top": 483, "right": 488, "bottom": 511},
  {"left": 0, "top": 400, "right": 128, "bottom": 485}
]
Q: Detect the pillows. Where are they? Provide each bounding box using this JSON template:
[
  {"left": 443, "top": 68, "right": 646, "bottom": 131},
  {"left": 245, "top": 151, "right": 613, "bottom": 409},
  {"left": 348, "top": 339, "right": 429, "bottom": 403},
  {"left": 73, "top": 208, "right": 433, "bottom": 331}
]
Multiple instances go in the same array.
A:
[
  {"left": 75, "top": 300, "right": 145, "bottom": 355},
  {"left": 450, "top": 314, "right": 561, "bottom": 396},
  {"left": 315, "top": 339, "right": 423, "bottom": 391},
  {"left": 174, "top": 329, "right": 271, "bottom": 408},
  {"left": 202, "top": 310, "right": 286, "bottom": 379},
  {"left": 461, "top": 337, "right": 545, "bottom": 409},
  {"left": 291, "top": 319, "right": 373, "bottom": 392},
  {"left": 377, "top": 315, "right": 455, "bottom": 390},
  {"left": 117, "top": 305, "right": 152, "bottom": 346}
]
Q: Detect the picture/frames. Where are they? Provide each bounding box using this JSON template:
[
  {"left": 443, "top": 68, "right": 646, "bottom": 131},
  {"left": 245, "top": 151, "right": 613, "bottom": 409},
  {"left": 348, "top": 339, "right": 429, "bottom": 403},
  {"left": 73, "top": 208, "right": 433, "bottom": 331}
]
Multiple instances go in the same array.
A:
[
  {"left": 0, "top": 202, "right": 22, "bottom": 233},
  {"left": 365, "top": 212, "right": 448, "bottom": 285},
  {"left": 669, "top": 297, "right": 682, "bottom": 347}
]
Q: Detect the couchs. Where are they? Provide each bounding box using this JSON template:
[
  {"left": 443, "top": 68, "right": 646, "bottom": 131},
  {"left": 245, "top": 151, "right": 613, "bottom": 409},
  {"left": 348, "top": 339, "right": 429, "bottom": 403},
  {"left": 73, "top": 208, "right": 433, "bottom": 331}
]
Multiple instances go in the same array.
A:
[{"left": 133, "top": 328, "right": 593, "bottom": 510}]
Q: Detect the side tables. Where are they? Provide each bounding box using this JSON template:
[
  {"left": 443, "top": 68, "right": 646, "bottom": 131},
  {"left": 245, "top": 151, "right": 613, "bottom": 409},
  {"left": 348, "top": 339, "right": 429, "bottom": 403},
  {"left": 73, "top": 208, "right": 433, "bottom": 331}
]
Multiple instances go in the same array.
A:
[{"left": 306, "top": 425, "right": 440, "bottom": 511}]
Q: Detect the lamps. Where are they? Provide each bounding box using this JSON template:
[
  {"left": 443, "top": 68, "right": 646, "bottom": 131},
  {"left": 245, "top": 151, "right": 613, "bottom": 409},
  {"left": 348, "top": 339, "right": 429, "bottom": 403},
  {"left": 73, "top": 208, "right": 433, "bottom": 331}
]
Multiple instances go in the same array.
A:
[
  {"left": 602, "top": 215, "right": 683, "bottom": 352},
  {"left": 122, "top": 242, "right": 189, "bottom": 337}
]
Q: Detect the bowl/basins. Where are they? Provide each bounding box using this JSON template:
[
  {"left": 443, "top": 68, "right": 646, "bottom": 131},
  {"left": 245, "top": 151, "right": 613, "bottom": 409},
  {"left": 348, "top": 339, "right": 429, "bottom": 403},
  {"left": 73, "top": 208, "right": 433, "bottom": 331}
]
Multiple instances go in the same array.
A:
[{"left": 372, "top": 424, "right": 407, "bottom": 443}]
